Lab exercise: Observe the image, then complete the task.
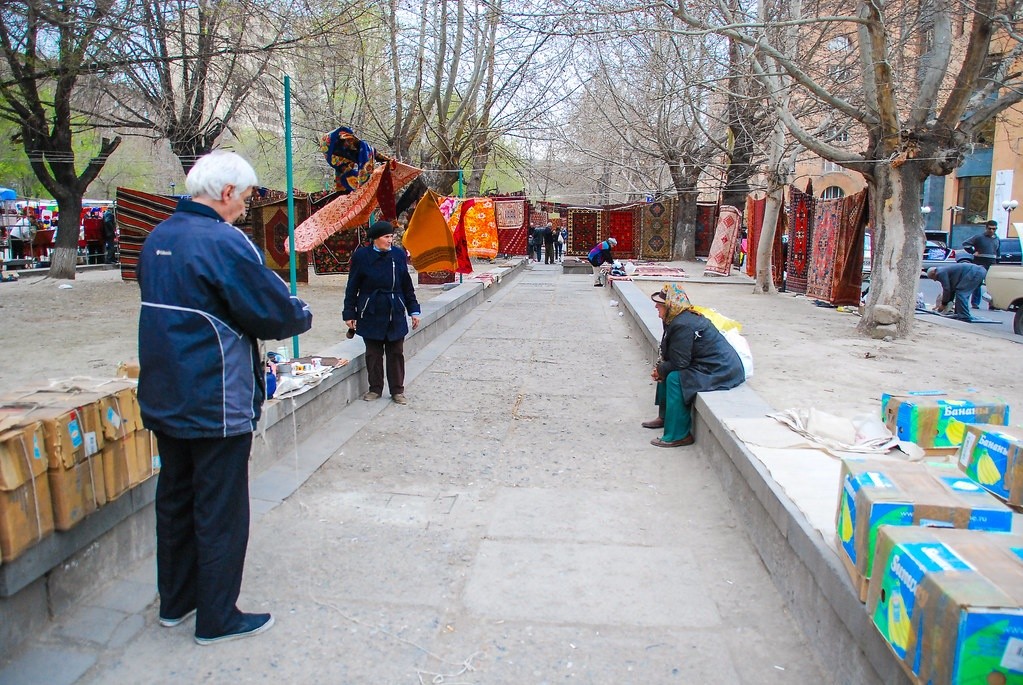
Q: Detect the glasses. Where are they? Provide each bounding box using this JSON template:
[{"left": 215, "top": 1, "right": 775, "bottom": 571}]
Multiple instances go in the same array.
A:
[{"left": 987, "top": 226, "right": 998, "bottom": 232}]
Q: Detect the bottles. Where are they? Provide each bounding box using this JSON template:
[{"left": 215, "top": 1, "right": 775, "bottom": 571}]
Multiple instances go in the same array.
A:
[{"left": 277, "top": 347, "right": 290, "bottom": 363}]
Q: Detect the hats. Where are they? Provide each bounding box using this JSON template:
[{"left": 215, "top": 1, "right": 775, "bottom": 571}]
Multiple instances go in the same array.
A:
[
  {"left": 367, "top": 220, "right": 395, "bottom": 240},
  {"left": 652, "top": 284, "right": 667, "bottom": 304}
]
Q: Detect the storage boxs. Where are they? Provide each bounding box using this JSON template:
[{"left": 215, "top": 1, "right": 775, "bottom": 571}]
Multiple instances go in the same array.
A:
[
  {"left": 0, "top": 362, "right": 162, "bottom": 563},
  {"left": 881, "top": 391, "right": 1009, "bottom": 457},
  {"left": 835, "top": 458, "right": 1012, "bottom": 603},
  {"left": 958, "top": 423, "right": 1023, "bottom": 514},
  {"left": 865, "top": 525, "right": 1023, "bottom": 685}
]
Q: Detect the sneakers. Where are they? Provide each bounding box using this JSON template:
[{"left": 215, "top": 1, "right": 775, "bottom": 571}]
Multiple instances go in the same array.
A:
[
  {"left": 650, "top": 433, "right": 696, "bottom": 448},
  {"left": 193, "top": 609, "right": 275, "bottom": 646},
  {"left": 641, "top": 416, "right": 667, "bottom": 429},
  {"left": 157, "top": 607, "right": 195, "bottom": 627}
]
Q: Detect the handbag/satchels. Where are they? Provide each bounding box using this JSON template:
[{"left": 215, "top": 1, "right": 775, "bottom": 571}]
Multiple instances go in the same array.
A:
[
  {"left": 557, "top": 231, "right": 564, "bottom": 244},
  {"left": 528, "top": 235, "right": 534, "bottom": 245}
]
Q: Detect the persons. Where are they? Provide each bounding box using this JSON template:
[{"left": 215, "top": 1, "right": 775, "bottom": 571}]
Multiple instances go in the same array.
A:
[
  {"left": 641, "top": 283, "right": 746, "bottom": 448},
  {"left": 84, "top": 212, "right": 103, "bottom": 265},
  {"left": 134, "top": 149, "right": 313, "bottom": 646},
  {"left": 962, "top": 220, "right": 1003, "bottom": 310},
  {"left": 529, "top": 222, "right": 567, "bottom": 264},
  {"left": 587, "top": 238, "right": 618, "bottom": 287},
  {"left": 927, "top": 262, "right": 987, "bottom": 321},
  {"left": 0, "top": 226, "right": 7, "bottom": 237},
  {"left": 5, "top": 215, "right": 36, "bottom": 270},
  {"left": 101, "top": 204, "right": 117, "bottom": 264},
  {"left": 341, "top": 221, "right": 421, "bottom": 404}
]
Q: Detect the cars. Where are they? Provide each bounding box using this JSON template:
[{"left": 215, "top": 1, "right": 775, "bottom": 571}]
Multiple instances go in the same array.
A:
[
  {"left": 922, "top": 223, "right": 988, "bottom": 281},
  {"left": 862, "top": 232, "right": 872, "bottom": 274},
  {"left": 995, "top": 237, "right": 1023, "bottom": 264}
]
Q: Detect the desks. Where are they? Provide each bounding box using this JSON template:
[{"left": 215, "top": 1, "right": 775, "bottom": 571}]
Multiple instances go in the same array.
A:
[{"left": 23, "top": 240, "right": 88, "bottom": 269}]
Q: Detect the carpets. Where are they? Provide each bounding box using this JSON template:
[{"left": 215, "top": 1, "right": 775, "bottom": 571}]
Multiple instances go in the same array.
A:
[{"left": 117, "top": 126, "right": 869, "bottom": 306}]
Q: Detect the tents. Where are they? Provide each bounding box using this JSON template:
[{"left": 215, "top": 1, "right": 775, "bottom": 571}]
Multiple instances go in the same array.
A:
[{"left": 0, "top": 186, "right": 117, "bottom": 211}]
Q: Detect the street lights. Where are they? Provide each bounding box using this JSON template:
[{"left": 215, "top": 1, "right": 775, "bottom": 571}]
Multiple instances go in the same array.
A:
[
  {"left": 1002, "top": 200, "right": 1019, "bottom": 238},
  {"left": 171, "top": 182, "right": 175, "bottom": 196}
]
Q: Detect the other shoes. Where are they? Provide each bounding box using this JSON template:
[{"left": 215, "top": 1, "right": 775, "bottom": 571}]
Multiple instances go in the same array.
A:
[
  {"left": 364, "top": 391, "right": 379, "bottom": 401},
  {"left": 392, "top": 393, "right": 407, "bottom": 405},
  {"left": 971, "top": 305, "right": 979, "bottom": 309},
  {"left": 594, "top": 283, "right": 603, "bottom": 287}
]
